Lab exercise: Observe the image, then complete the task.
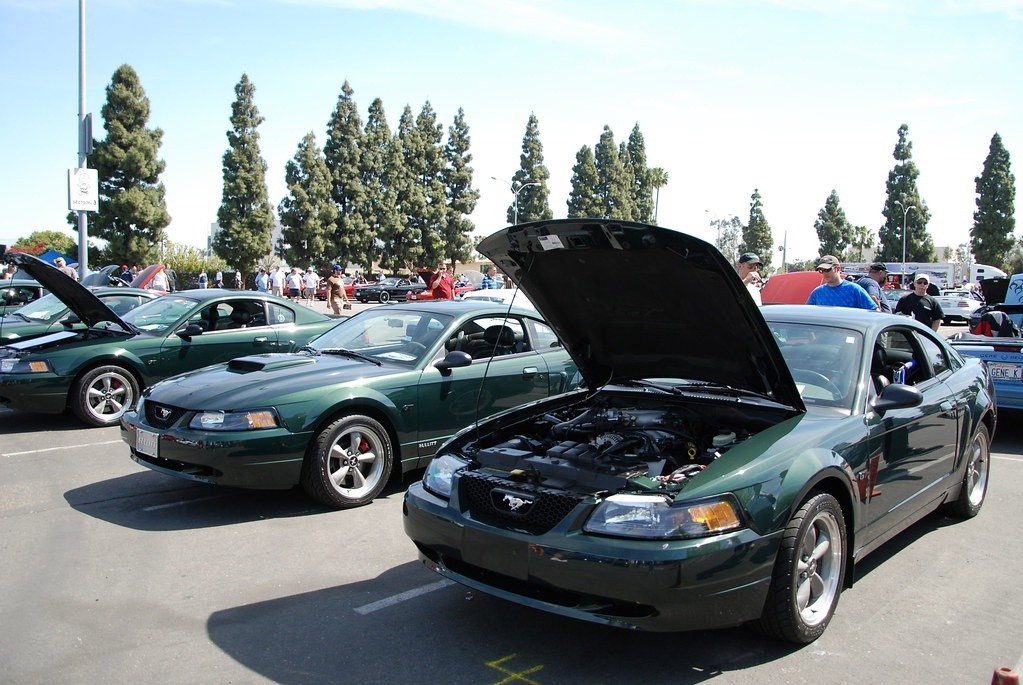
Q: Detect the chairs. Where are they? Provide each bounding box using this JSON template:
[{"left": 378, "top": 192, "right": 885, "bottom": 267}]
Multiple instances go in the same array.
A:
[
  {"left": 471, "top": 326, "right": 515, "bottom": 360},
  {"left": 229, "top": 308, "right": 255, "bottom": 329},
  {"left": 194, "top": 308, "right": 220, "bottom": 332},
  {"left": 836, "top": 341, "right": 891, "bottom": 386},
  {"left": 414, "top": 332, "right": 453, "bottom": 361}
]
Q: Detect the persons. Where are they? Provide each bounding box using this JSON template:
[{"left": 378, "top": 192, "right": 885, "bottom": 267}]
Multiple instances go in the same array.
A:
[
  {"left": 355, "top": 271, "right": 369, "bottom": 284},
  {"left": 121, "top": 263, "right": 177, "bottom": 291},
  {"left": 738, "top": 252, "right": 762, "bottom": 306},
  {"left": 270, "top": 265, "right": 285, "bottom": 297},
  {"left": 409, "top": 274, "right": 423, "bottom": 283},
  {"left": 55, "top": 257, "right": 78, "bottom": 282},
  {"left": 4, "top": 266, "right": 16, "bottom": 279},
  {"left": 962, "top": 280, "right": 979, "bottom": 293},
  {"left": 286, "top": 269, "right": 303, "bottom": 304},
  {"left": 326, "top": 265, "right": 349, "bottom": 315},
  {"left": 429, "top": 263, "right": 466, "bottom": 299},
  {"left": 482, "top": 266, "right": 497, "bottom": 301},
  {"left": 255, "top": 270, "right": 271, "bottom": 292},
  {"left": 893, "top": 274, "right": 945, "bottom": 348},
  {"left": 301, "top": 267, "right": 319, "bottom": 307},
  {"left": 214, "top": 269, "right": 222, "bottom": 288},
  {"left": 198, "top": 269, "right": 208, "bottom": 289},
  {"left": 235, "top": 269, "right": 242, "bottom": 289},
  {"left": 376, "top": 271, "right": 386, "bottom": 281},
  {"left": 805, "top": 256, "right": 894, "bottom": 347}
]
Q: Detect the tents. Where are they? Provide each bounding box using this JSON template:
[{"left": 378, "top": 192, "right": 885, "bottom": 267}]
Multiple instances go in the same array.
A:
[{"left": 37, "top": 249, "right": 77, "bottom": 266}]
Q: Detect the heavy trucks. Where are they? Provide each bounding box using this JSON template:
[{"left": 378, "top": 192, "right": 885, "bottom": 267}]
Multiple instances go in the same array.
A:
[{"left": 838, "top": 262, "right": 1008, "bottom": 291}]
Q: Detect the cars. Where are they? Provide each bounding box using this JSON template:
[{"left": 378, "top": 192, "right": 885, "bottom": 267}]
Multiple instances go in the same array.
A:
[
  {"left": 461, "top": 289, "right": 536, "bottom": 312},
  {"left": 882, "top": 290, "right": 913, "bottom": 314},
  {"left": 403, "top": 218, "right": 998, "bottom": 643},
  {"left": 406, "top": 269, "right": 474, "bottom": 301},
  {"left": 119, "top": 300, "right": 585, "bottom": 508},
  {"left": 0, "top": 265, "right": 169, "bottom": 315},
  {"left": 352, "top": 277, "right": 429, "bottom": 304},
  {"left": 0, "top": 286, "right": 162, "bottom": 342},
  {"left": 761, "top": 271, "right": 848, "bottom": 305},
  {"left": 0, "top": 252, "right": 352, "bottom": 427}
]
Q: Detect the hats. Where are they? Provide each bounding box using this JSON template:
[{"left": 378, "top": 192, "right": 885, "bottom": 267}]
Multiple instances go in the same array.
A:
[
  {"left": 261, "top": 269, "right": 266, "bottom": 272},
  {"left": 915, "top": 273, "right": 930, "bottom": 283},
  {"left": 122, "top": 265, "right": 127, "bottom": 270},
  {"left": 333, "top": 265, "right": 344, "bottom": 271},
  {"left": 870, "top": 261, "right": 890, "bottom": 273},
  {"left": 739, "top": 253, "right": 764, "bottom": 267},
  {"left": 962, "top": 279, "right": 968, "bottom": 282},
  {"left": 816, "top": 254, "right": 840, "bottom": 270},
  {"left": 308, "top": 267, "right": 313, "bottom": 271}
]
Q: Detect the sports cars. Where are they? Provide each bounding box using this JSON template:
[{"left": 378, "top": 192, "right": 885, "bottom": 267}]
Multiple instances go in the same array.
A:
[
  {"left": 929, "top": 289, "right": 985, "bottom": 325},
  {"left": 945, "top": 305, "right": 1023, "bottom": 409},
  {"left": 284, "top": 277, "right": 376, "bottom": 300}
]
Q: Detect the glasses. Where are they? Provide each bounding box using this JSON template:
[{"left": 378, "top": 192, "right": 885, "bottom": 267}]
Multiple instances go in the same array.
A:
[
  {"left": 818, "top": 265, "right": 837, "bottom": 273},
  {"left": 439, "top": 268, "right": 446, "bottom": 270},
  {"left": 914, "top": 281, "right": 928, "bottom": 285}
]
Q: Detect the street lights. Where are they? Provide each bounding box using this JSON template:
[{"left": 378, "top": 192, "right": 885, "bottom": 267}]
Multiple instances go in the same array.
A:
[{"left": 491, "top": 176, "right": 541, "bottom": 225}]
